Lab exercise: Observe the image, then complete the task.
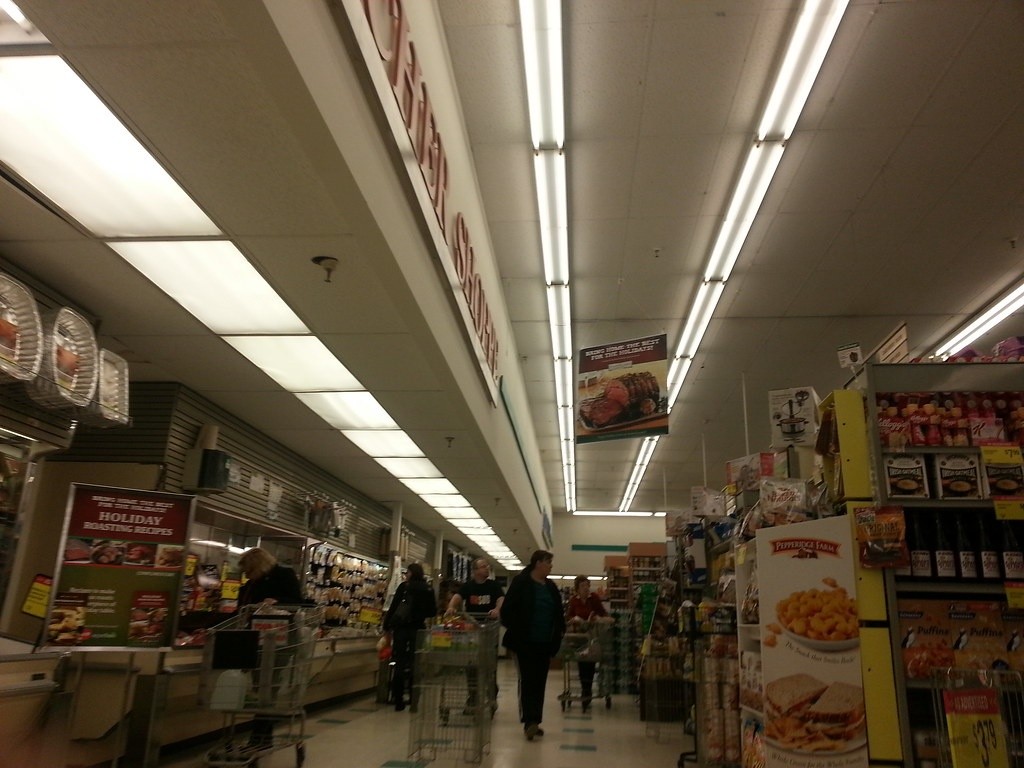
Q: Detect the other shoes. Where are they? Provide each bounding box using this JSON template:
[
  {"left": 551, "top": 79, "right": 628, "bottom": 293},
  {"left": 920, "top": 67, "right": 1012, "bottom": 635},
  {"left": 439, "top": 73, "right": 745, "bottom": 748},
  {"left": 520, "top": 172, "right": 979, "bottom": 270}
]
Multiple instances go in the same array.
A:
[
  {"left": 524, "top": 723, "right": 538, "bottom": 740},
  {"left": 463, "top": 698, "right": 476, "bottom": 715},
  {"left": 396, "top": 703, "right": 405, "bottom": 710},
  {"left": 536, "top": 728, "right": 542, "bottom": 736},
  {"left": 581, "top": 692, "right": 592, "bottom": 708},
  {"left": 240, "top": 740, "right": 272, "bottom": 753}
]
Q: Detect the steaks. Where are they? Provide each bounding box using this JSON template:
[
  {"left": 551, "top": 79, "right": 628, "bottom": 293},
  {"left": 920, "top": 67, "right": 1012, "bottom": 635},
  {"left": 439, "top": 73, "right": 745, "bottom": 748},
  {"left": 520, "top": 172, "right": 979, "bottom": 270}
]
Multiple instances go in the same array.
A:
[{"left": 579, "top": 372, "right": 660, "bottom": 427}]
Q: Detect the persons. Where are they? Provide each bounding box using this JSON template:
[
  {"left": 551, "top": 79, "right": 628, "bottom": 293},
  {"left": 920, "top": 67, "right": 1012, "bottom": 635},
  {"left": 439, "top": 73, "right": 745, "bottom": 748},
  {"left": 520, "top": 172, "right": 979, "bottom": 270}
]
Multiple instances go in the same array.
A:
[
  {"left": 381, "top": 563, "right": 435, "bottom": 713},
  {"left": 444, "top": 559, "right": 505, "bottom": 717},
  {"left": 498, "top": 550, "right": 565, "bottom": 739},
  {"left": 563, "top": 574, "right": 609, "bottom": 709},
  {"left": 231, "top": 546, "right": 303, "bottom": 754}
]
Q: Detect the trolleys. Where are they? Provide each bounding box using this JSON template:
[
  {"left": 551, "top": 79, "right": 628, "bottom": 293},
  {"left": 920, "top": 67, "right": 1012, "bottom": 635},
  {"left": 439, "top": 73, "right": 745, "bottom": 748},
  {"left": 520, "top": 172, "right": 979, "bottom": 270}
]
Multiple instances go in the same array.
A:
[
  {"left": 199, "top": 603, "right": 326, "bottom": 768},
  {"left": 409, "top": 612, "right": 500, "bottom": 766},
  {"left": 558, "top": 625, "right": 614, "bottom": 713}
]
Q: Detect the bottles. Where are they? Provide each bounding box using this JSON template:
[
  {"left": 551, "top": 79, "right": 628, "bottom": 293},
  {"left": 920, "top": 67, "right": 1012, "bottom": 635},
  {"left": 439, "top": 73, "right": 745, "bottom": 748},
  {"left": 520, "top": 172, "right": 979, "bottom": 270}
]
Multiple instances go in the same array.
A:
[{"left": 889, "top": 510, "right": 1022, "bottom": 582}]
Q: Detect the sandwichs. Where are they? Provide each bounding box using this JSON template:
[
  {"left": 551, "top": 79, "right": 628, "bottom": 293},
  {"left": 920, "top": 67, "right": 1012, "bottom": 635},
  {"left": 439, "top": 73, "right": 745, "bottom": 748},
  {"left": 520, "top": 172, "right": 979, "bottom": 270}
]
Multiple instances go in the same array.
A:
[{"left": 767, "top": 675, "right": 865, "bottom": 741}]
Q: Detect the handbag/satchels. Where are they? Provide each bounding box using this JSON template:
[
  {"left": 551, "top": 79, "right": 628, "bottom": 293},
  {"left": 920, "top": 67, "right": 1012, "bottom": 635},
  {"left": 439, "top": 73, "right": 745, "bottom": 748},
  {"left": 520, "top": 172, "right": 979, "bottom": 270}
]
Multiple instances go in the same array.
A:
[{"left": 390, "top": 582, "right": 412, "bottom": 627}]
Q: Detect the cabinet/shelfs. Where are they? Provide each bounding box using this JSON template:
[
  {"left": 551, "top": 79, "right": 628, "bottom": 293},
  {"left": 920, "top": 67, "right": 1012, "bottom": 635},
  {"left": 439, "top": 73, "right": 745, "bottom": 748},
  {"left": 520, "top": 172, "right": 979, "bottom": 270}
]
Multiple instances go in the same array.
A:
[
  {"left": 171, "top": 497, "right": 395, "bottom": 630},
  {"left": 593, "top": 352, "right": 1024, "bottom": 768}
]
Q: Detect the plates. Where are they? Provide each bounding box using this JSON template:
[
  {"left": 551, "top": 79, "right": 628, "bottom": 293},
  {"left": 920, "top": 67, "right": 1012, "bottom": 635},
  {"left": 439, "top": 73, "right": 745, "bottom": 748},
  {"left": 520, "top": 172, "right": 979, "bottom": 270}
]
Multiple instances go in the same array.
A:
[
  {"left": 776, "top": 613, "right": 860, "bottom": 652},
  {"left": 579, "top": 409, "right": 666, "bottom": 431},
  {"left": 765, "top": 735, "right": 868, "bottom": 757}
]
